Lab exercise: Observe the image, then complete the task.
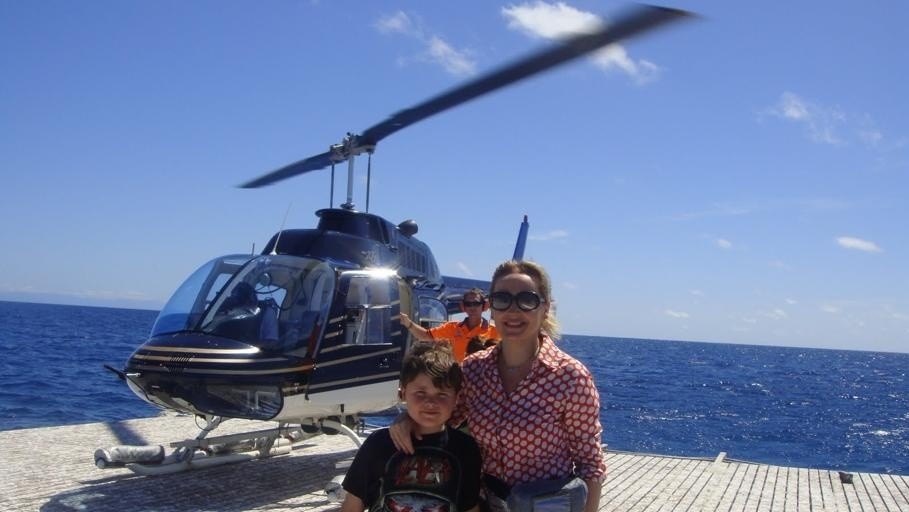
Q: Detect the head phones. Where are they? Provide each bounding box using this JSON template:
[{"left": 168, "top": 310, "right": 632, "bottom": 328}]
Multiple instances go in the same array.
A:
[{"left": 460, "top": 298, "right": 490, "bottom": 311}]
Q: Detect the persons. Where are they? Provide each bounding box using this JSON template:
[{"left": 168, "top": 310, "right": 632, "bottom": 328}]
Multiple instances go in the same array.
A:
[
  {"left": 397, "top": 284, "right": 501, "bottom": 369},
  {"left": 215, "top": 281, "right": 280, "bottom": 351},
  {"left": 463, "top": 334, "right": 498, "bottom": 359},
  {"left": 387, "top": 260, "right": 608, "bottom": 512},
  {"left": 336, "top": 337, "right": 488, "bottom": 512}
]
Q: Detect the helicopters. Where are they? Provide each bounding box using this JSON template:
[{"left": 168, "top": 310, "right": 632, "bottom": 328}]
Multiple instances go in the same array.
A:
[{"left": 95, "top": 3, "right": 704, "bottom": 505}]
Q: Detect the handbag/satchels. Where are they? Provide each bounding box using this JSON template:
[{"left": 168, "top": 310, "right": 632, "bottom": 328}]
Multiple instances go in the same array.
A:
[{"left": 505, "top": 473, "right": 588, "bottom": 512}]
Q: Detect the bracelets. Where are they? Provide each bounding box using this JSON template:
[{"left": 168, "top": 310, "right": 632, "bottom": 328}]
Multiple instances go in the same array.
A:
[{"left": 407, "top": 320, "right": 414, "bottom": 330}]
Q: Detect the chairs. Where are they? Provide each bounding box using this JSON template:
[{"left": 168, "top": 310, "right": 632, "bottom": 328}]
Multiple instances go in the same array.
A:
[{"left": 290, "top": 310, "right": 320, "bottom": 347}]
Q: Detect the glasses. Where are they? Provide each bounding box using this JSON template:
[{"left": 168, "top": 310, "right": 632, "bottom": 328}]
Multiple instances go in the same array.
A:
[
  {"left": 462, "top": 300, "right": 483, "bottom": 307},
  {"left": 485, "top": 290, "right": 548, "bottom": 313}
]
